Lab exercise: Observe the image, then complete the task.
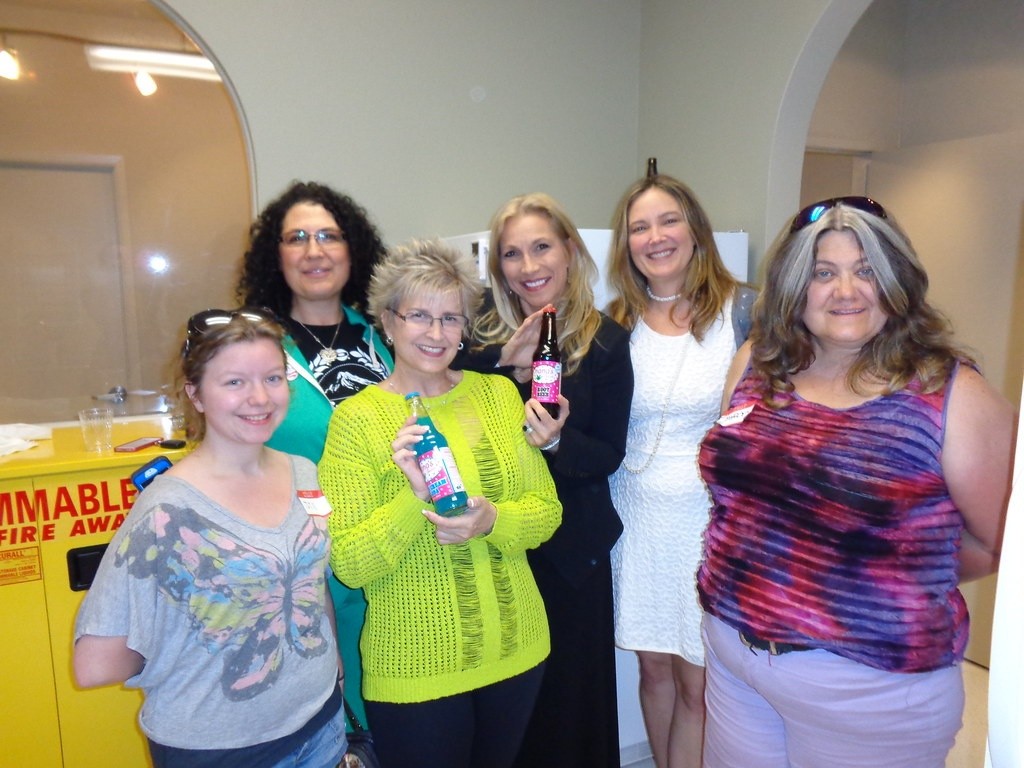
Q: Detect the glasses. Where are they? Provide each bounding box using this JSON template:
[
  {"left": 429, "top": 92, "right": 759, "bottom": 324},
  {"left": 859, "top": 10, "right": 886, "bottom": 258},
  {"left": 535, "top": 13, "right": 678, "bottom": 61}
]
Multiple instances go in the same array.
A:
[
  {"left": 176, "top": 307, "right": 287, "bottom": 343},
  {"left": 382, "top": 300, "right": 477, "bottom": 331},
  {"left": 777, "top": 192, "right": 908, "bottom": 229},
  {"left": 278, "top": 228, "right": 353, "bottom": 251}
]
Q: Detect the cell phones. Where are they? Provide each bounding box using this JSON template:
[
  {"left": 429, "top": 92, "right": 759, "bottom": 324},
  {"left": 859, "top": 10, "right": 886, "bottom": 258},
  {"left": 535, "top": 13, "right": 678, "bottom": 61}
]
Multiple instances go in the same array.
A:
[
  {"left": 115, "top": 437, "right": 164, "bottom": 452},
  {"left": 131, "top": 455, "right": 173, "bottom": 493}
]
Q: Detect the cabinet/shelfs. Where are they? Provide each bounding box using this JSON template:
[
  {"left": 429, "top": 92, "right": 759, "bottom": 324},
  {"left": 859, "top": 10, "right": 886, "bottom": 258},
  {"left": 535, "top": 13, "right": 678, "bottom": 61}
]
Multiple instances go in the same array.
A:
[{"left": 0, "top": 420, "right": 200, "bottom": 768}]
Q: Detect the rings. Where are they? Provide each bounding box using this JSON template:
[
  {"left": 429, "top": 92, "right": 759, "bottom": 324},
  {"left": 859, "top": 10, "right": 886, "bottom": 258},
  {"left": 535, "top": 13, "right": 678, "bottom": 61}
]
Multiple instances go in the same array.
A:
[{"left": 523, "top": 426, "right": 534, "bottom": 435}]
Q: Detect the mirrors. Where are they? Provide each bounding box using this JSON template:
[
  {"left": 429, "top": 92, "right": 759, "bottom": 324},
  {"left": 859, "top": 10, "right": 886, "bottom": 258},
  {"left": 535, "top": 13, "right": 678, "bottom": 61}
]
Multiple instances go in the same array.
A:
[{"left": 0, "top": 0, "right": 259, "bottom": 424}]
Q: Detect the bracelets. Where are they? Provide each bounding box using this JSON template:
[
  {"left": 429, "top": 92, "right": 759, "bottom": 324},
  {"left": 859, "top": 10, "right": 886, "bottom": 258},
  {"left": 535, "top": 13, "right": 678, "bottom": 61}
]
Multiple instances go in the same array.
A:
[
  {"left": 540, "top": 439, "right": 560, "bottom": 450},
  {"left": 496, "top": 363, "right": 499, "bottom": 368}
]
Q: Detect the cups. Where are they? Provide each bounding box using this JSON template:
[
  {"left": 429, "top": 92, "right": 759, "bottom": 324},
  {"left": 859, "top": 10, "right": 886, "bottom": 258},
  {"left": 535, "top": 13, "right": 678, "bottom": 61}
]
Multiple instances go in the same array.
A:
[{"left": 77, "top": 410, "right": 117, "bottom": 455}]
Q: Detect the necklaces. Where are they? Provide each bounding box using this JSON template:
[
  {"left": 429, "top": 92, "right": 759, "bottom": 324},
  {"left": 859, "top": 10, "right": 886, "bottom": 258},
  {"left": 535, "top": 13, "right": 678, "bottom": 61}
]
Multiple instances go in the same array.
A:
[
  {"left": 646, "top": 285, "right": 682, "bottom": 302},
  {"left": 290, "top": 307, "right": 343, "bottom": 362},
  {"left": 386, "top": 369, "right": 454, "bottom": 408},
  {"left": 623, "top": 335, "right": 691, "bottom": 474}
]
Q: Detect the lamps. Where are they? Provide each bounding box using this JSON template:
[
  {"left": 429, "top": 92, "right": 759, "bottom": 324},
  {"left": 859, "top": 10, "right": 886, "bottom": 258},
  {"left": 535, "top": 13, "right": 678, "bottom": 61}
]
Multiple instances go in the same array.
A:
[{"left": 85, "top": 44, "right": 223, "bottom": 84}]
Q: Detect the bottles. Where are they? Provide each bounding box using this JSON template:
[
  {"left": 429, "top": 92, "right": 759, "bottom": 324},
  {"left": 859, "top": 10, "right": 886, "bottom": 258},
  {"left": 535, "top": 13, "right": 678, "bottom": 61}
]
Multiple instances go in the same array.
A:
[
  {"left": 405, "top": 393, "right": 470, "bottom": 518},
  {"left": 531, "top": 307, "right": 562, "bottom": 421}
]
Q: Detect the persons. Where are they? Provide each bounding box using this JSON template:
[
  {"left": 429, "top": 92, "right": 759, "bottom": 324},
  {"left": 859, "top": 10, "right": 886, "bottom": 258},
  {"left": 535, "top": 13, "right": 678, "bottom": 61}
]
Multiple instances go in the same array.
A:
[
  {"left": 318, "top": 239, "right": 562, "bottom": 767},
  {"left": 75, "top": 311, "right": 348, "bottom": 766},
  {"left": 601, "top": 176, "right": 764, "bottom": 768},
  {"left": 234, "top": 180, "right": 395, "bottom": 768},
  {"left": 700, "top": 195, "right": 1017, "bottom": 768},
  {"left": 449, "top": 194, "right": 635, "bottom": 768}
]
